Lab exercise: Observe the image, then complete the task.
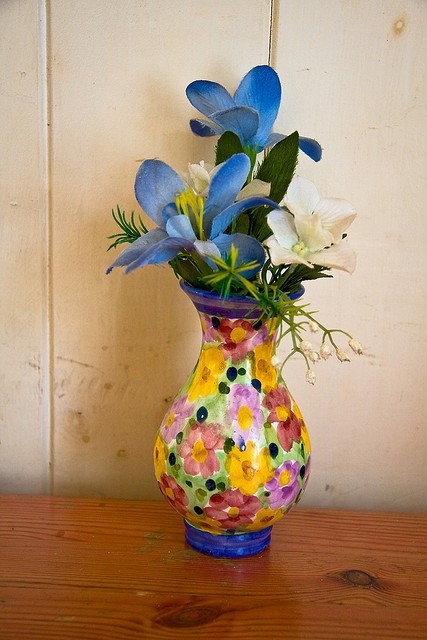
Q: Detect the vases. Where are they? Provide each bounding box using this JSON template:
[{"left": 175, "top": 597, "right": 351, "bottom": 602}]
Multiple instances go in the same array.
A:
[{"left": 152, "top": 276, "right": 313, "bottom": 560}]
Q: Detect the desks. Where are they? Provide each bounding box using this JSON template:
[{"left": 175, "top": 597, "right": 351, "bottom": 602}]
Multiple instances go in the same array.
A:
[{"left": 0, "top": 491, "right": 427, "bottom": 639}]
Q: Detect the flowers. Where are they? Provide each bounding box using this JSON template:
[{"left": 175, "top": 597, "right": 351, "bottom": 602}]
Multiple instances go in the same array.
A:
[{"left": 105, "top": 62, "right": 367, "bottom": 392}]
